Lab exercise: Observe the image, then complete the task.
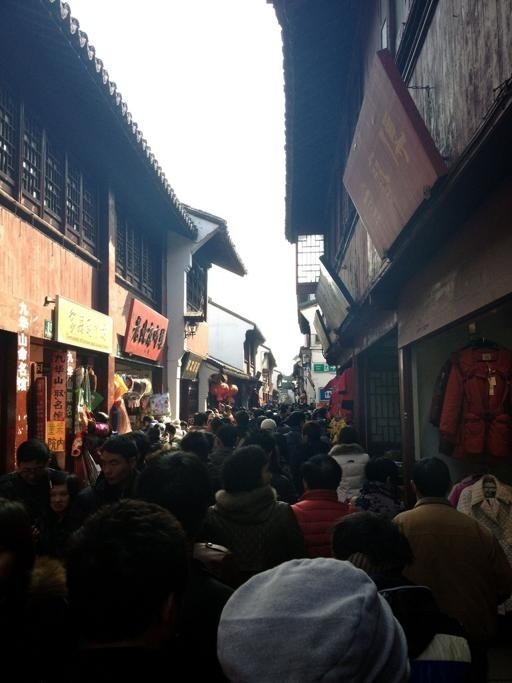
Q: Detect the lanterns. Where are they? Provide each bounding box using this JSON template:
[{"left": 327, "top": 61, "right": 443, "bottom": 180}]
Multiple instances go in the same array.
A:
[
  {"left": 226, "top": 384, "right": 238, "bottom": 400},
  {"left": 210, "top": 382, "right": 229, "bottom": 401}
]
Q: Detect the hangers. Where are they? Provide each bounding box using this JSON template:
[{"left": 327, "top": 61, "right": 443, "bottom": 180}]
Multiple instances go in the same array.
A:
[{"left": 462, "top": 338, "right": 496, "bottom": 350}]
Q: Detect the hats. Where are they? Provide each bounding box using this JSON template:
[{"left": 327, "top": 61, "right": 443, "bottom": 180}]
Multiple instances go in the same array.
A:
[
  {"left": 260, "top": 417, "right": 277, "bottom": 431},
  {"left": 217, "top": 556, "right": 409, "bottom": 682}
]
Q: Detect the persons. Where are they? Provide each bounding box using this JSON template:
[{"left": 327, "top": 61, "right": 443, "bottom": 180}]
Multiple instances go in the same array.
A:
[{"left": 1, "top": 400, "right": 510, "bottom": 682}]
